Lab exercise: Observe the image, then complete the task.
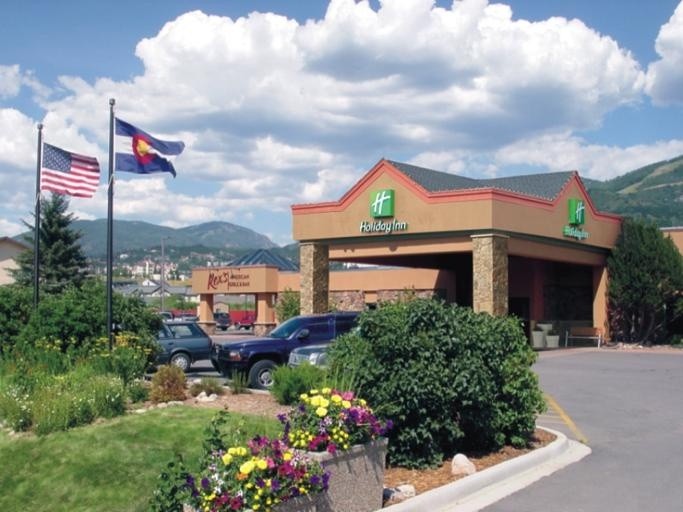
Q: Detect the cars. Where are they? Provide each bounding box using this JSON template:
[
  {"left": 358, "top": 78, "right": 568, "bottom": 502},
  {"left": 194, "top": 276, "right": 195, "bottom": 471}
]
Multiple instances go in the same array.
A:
[{"left": 157, "top": 311, "right": 361, "bottom": 390}]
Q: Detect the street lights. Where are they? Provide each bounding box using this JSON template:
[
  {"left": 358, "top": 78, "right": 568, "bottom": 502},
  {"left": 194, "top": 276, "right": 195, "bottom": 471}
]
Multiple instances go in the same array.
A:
[{"left": 161, "top": 236, "right": 169, "bottom": 311}]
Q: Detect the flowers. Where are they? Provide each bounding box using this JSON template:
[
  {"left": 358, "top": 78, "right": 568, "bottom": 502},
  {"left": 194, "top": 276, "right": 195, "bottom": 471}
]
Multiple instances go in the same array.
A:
[{"left": 150, "top": 385, "right": 394, "bottom": 512}]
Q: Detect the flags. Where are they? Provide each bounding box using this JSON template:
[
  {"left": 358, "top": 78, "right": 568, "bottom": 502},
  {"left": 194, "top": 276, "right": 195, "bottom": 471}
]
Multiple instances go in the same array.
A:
[
  {"left": 40, "top": 143, "right": 101, "bottom": 199},
  {"left": 114, "top": 118, "right": 185, "bottom": 188}
]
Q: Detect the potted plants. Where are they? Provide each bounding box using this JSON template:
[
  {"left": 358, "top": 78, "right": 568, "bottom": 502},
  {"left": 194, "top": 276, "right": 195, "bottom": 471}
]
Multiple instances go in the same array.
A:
[{"left": 531, "top": 319, "right": 561, "bottom": 349}]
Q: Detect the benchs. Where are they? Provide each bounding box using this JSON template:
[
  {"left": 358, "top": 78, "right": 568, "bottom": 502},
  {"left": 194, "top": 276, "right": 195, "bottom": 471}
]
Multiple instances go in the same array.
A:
[{"left": 564, "top": 327, "right": 604, "bottom": 351}]
